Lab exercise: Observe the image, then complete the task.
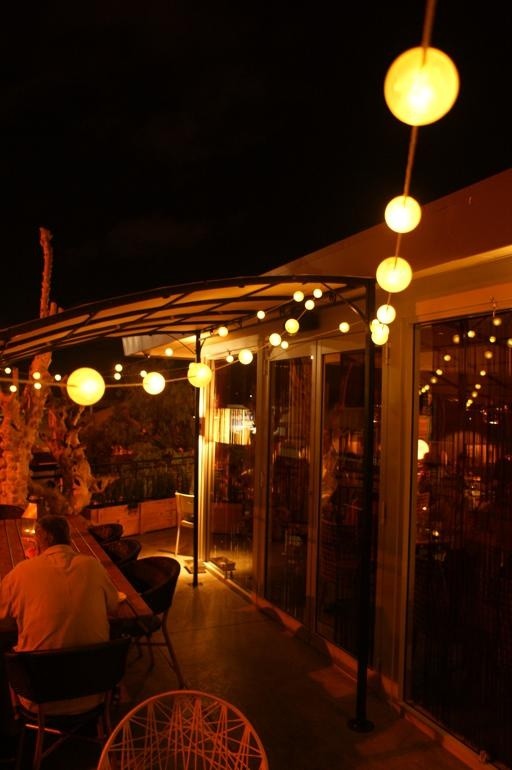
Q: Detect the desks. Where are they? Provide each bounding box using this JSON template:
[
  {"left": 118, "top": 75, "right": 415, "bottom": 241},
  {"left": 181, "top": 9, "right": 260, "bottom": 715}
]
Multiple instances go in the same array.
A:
[{"left": 0, "top": 506, "right": 153, "bottom": 638}]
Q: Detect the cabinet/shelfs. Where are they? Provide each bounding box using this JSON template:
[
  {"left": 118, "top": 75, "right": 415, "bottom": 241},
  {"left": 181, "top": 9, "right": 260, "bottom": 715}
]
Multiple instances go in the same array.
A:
[
  {"left": 139, "top": 498, "right": 177, "bottom": 535},
  {"left": 86, "top": 502, "right": 140, "bottom": 537}
]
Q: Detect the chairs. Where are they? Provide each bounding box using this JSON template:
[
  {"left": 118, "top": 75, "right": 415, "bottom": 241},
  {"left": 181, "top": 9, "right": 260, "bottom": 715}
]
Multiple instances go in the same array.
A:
[
  {"left": 101, "top": 537, "right": 142, "bottom": 565},
  {"left": 124, "top": 556, "right": 181, "bottom": 681},
  {"left": 97, "top": 690, "right": 269, "bottom": 770},
  {"left": 87, "top": 523, "right": 123, "bottom": 545},
  {"left": 3, "top": 634, "right": 131, "bottom": 770},
  {"left": 282, "top": 491, "right": 452, "bottom": 649},
  {"left": 175, "top": 492, "right": 195, "bottom": 555}
]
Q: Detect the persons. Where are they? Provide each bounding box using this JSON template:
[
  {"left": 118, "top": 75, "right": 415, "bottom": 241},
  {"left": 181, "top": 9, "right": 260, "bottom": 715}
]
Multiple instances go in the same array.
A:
[{"left": 0, "top": 516, "right": 120, "bottom": 770}]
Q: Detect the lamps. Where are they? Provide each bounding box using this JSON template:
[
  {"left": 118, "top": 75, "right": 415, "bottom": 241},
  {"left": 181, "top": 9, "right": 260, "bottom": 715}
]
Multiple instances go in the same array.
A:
[{"left": 21, "top": 503, "right": 37, "bottom": 537}]
